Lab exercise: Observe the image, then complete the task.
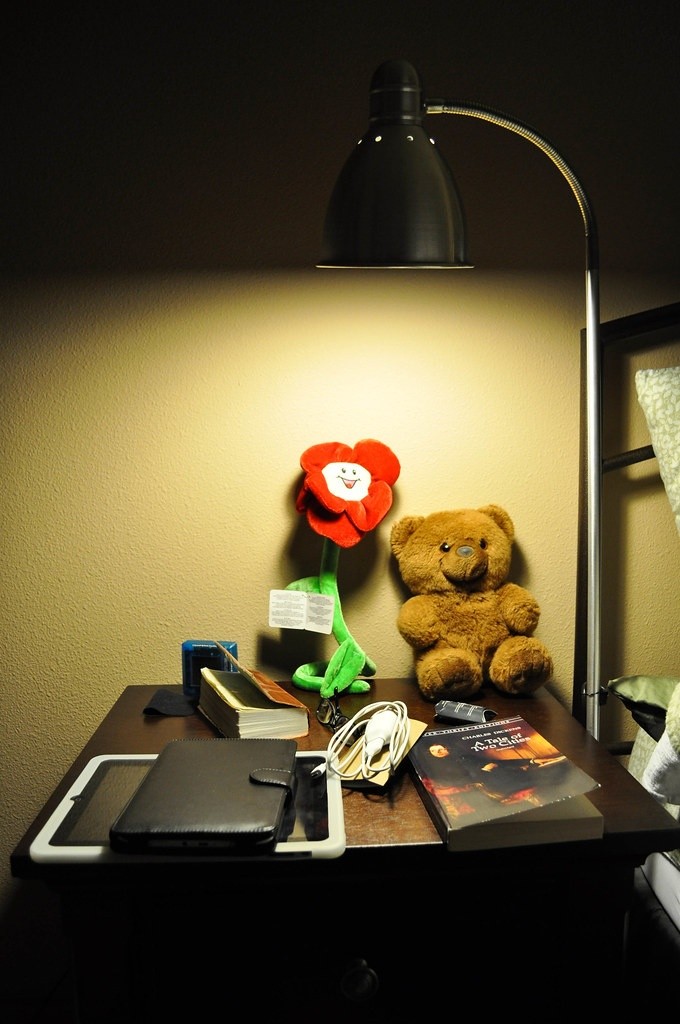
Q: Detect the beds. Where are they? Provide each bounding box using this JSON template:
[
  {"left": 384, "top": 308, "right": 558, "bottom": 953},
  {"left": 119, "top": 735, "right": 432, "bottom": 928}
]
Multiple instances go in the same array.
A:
[{"left": 571, "top": 299, "right": 680, "bottom": 1024}]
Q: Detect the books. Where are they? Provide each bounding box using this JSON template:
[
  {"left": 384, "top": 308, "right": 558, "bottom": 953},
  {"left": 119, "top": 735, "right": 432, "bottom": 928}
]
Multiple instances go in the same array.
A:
[
  {"left": 405, "top": 715, "right": 605, "bottom": 851},
  {"left": 109, "top": 739, "right": 299, "bottom": 851},
  {"left": 198, "top": 642, "right": 310, "bottom": 740}
]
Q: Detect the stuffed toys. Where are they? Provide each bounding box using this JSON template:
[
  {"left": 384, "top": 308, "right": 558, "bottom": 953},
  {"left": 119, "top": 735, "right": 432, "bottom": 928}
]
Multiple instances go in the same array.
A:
[
  {"left": 281, "top": 439, "right": 401, "bottom": 698},
  {"left": 390, "top": 505, "right": 553, "bottom": 699}
]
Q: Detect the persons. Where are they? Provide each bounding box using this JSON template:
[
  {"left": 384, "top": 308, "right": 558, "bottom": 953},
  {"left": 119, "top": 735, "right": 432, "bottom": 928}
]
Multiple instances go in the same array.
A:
[{"left": 428, "top": 742, "right": 562, "bottom": 806}]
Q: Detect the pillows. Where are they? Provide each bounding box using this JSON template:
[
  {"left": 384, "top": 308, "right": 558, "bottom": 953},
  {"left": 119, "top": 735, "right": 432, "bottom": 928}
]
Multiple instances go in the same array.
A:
[
  {"left": 635, "top": 367, "right": 680, "bottom": 536},
  {"left": 608, "top": 675, "right": 680, "bottom": 743}
]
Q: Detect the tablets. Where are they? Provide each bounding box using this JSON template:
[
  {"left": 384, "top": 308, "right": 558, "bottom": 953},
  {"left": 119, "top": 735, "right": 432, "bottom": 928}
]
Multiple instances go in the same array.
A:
[{"left": 30, "top": 750, "right": 346, "bottom": 863}]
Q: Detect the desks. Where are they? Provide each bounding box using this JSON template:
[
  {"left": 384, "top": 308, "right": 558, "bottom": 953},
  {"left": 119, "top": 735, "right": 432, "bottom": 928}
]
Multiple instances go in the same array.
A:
[{"left": 0, "top": 676, "right": 680, "bottom": 1024}]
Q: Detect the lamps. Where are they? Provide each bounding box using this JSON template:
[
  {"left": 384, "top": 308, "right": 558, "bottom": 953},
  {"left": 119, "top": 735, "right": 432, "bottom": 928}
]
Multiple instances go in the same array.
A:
[{"left": 315, "top": 57, "right": 607, "bottom": 739}]
